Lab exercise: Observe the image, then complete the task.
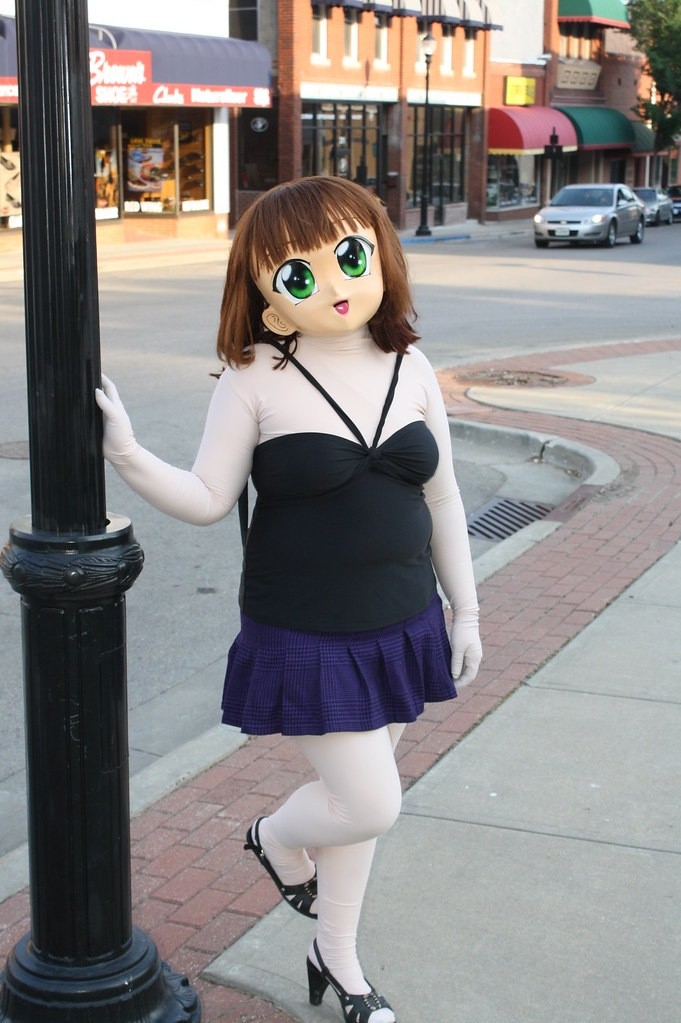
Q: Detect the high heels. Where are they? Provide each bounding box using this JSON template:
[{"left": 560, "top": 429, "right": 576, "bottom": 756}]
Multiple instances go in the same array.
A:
[
  {"left": 305, "top": 938, "right": 396, "bottom": 1022},
  {"left": 241, "top": 816, "right": 326, "bottom": 918}
]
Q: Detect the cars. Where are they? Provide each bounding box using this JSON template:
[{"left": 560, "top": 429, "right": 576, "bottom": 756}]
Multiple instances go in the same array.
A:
[
  {"left": 632, "top": 185, "right": 680, "bottom": 226},
  {"left": 533, "top": 183, "right": 644, "bottom": 248}
]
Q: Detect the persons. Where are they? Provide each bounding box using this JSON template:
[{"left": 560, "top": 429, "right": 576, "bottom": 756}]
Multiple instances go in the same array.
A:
[
  {"left": 93, "top": 177, "right": 484, "bottom": 1022},
  {"left": 578, "top": 191, "right": 612, "bottom": 207}
]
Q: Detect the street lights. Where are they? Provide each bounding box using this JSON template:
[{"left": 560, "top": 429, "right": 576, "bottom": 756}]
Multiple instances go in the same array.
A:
[{"left": 415, "top": 31, "right": 437, "bottom": 236}]
